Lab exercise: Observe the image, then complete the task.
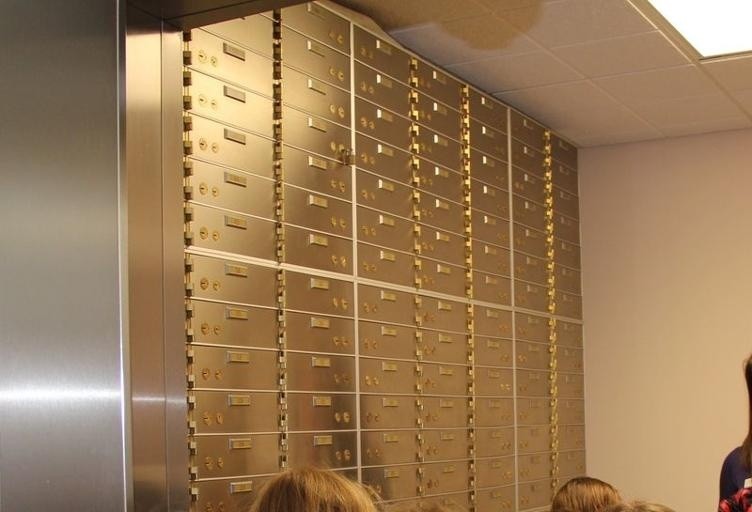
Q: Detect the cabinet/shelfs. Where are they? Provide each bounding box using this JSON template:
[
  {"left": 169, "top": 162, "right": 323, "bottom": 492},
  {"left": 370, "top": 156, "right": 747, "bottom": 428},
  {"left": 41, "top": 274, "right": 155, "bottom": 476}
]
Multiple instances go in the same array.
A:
[{"left": 127, "top": 1, "right": 588, "bottom": 512}]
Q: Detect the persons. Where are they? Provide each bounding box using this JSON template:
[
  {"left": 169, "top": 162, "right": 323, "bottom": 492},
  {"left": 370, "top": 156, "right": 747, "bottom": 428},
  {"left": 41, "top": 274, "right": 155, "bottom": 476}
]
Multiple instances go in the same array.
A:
[
  {"left": 250, "top": 467, "right": 385, "bottom": 512},
  {"left": 604, "top": 498, "right": 672, "bottom": 512},
  {"left": 552, "top": 477, "right": 621, "bottom": 512},
  {"left": 719, "top": 354, "right": 752, "bottom": 512}
]
